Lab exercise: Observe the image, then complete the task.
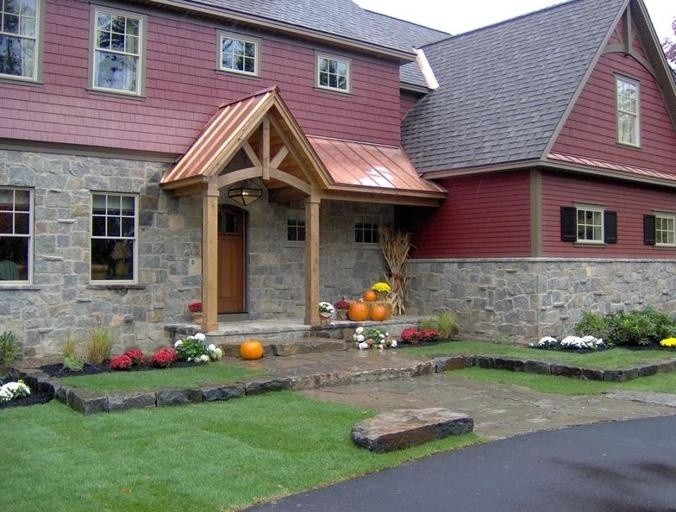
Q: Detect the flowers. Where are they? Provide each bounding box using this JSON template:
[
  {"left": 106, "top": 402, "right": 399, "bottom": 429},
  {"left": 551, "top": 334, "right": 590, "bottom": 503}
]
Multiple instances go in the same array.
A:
[
  {"left": 319, "top": 301, "right": 334, "bottom": 314},
  {"left": 334, "top": 300, "right": 350, "bottom": 310}
]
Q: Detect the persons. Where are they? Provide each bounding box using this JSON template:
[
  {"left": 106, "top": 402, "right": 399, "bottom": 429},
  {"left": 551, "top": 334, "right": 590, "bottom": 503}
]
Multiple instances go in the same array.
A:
[{"left": 0, "top": 249, "right": 19, "bottom": 280}]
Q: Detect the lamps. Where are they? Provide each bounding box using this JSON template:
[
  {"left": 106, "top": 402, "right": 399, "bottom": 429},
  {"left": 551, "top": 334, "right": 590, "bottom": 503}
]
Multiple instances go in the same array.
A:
[
  {"left": 227, "top": 155, "right": 261, "bottom": 206},
  {"left": 111, "top": 241, "right": 131, "bottom": 276}
]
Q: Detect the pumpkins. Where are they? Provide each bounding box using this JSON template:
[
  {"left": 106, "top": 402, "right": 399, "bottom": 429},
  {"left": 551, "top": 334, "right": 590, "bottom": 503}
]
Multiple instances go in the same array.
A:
[
  {"left": 364, "top": 290, "right": 377, "bottom": 302},
  {"left": 349, "top": 303, "right": 368, "bottom": 320},
  {"left": 370, "top": 305, "right": 387, "bottom": 320},
  {"left": 240, "top": 340, "right": 263, "bottom": 360}
]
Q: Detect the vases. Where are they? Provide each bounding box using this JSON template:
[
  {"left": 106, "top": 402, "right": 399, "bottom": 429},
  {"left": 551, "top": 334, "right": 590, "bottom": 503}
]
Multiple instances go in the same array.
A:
[
  {"left": 335, "top": 309, "right": 349, "bottom": 320},
  {"left": 318, "top": 312, "right": 332, "bottom": 324}
]
